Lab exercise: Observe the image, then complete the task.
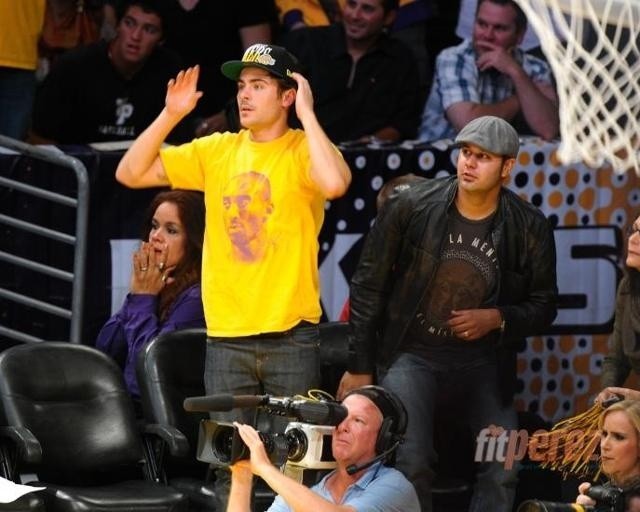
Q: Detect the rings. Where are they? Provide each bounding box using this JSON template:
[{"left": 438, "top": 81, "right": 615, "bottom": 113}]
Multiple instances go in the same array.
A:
[
  {"left": 160, "top": 262, "right": 165, "bottom": 271},
  {"left": 140, "top": 266, "right": 147, "bottom": 272},
  {"left": 464, "top": 330, "right": 469, "bottom": 338},
  {"left": 161, "top": 275, "right": 166, "bottom": 281}
]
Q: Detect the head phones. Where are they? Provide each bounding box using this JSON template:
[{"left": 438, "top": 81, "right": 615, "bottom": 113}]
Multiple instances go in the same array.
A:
[{"left": 340, "top": 385, "right": 407, "bottom": 454}]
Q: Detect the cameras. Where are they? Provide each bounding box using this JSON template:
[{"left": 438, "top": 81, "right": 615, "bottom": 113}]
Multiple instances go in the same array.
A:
[{"left": 516, "top": 486, "right": 624, "bottom": 512}]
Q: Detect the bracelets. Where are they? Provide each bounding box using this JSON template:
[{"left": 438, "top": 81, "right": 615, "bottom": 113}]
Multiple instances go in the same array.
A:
[{"left": 500, "top": 318, "right": 506, "bottom": 332}]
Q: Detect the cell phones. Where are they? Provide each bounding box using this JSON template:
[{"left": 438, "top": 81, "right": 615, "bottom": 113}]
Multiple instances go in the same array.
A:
[{"left": 601, "top": 395, "right": 624, "bottom": 408}]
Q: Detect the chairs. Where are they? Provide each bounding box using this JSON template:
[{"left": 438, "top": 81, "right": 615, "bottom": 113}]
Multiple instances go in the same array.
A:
[
  {"left": 319, "top": 323, "right": 479, "bottom": 499},
  {"left": 140, "top": 327, "right": 279, "bottom": 507},
  {"left": 0, "top": 342, "right": 195, "bottom": 511}
]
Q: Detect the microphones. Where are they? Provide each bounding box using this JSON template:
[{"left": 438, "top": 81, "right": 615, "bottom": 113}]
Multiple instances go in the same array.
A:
[{"left": 347, "top": 441, "right": 400, "bottom": 475}]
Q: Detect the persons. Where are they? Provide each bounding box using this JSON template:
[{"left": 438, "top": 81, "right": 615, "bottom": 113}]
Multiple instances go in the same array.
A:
[
  {"left": 0, "top": 1, "right": 561, "bottom": 142},
  {"left": 593, "top": 212, "right": 639, "bottom": 404},
  {"left": 336, "top": 172, "right": 430, "bottom": 317},
  {"left": 225, "top": 383, "right": 422, "bottom": 512},
  {"left": 573, "top": 398, "right": 639, "bottom": 512},
  {"left": 93, "top": 189, "right": 206, "bottom": 407},
  {"left": 219, "top": 170, "right": 280, "bottom": 262},
  {"left": 335, "top": 114, "right": 560, "bottom": 512},
  {"left": 116, "top": 42, "right": 354, "bottom": 511}
]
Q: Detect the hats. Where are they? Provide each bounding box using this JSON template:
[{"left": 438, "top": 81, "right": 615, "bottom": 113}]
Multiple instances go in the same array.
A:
[
  {"left": 454, "top": 115, "right": 519, "bottom": 158},
  {"left": 221, "top": 43, "right": 299, "bottom": 80}
]
